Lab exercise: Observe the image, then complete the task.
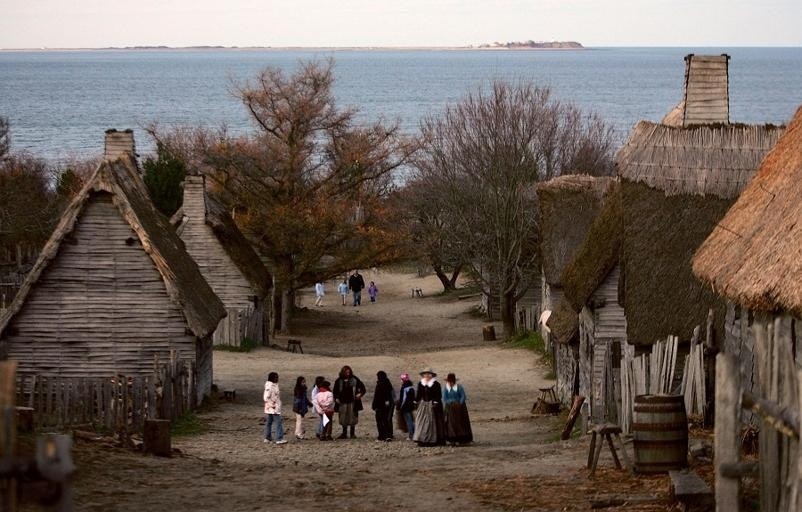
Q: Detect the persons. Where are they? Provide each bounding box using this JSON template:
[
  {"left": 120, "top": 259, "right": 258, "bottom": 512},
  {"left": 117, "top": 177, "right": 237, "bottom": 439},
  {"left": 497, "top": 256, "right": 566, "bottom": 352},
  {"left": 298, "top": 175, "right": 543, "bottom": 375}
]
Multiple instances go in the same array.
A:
[
  {"left": 367, "top": 281, "right": 378, "bottom": 304},
  {"left": 311, "top": 366, "right": 366, "bottom": 440},
  {"left": 294, "top": 377, "right": 313, "bottom": 439},
  {"left": 372, "top": 371, "right": 395, "bottom": 441},
  {"left": 348, "top": 270, "right": 365, "bottom": 307},
  {"left": 442, "top": 374, "right": 472, "bottom": 446},
  {"left": 338, "top": 280, "right": 350, "bottom": 305},
  {"left": 412, "top": 367, "right": 444, "bottom": 446},
  {"left": 263, "top": 372, "right": 288, "bottom": 445},
  {"left": 396, "top": 374, "right": 418, "bottom": 440},
  {"left": 315, "top": 281, "right": 324, "bottom": 307}
]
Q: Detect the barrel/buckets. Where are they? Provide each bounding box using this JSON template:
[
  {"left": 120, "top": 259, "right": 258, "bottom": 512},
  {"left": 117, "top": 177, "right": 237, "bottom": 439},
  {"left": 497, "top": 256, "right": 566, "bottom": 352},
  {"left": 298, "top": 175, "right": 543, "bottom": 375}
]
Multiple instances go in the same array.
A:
[{"left": 631, "top": 392, "right": 688, "bottom": 476}]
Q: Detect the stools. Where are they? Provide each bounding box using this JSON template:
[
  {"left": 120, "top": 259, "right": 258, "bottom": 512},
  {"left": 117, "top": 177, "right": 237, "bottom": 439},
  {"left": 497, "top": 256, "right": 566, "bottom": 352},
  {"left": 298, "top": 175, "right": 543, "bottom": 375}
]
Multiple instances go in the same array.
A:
[
  {"left": 286, "top": 339, "right": 304, "bottom": 353},
  {"left": 587, "top": 422, "right": 634, "bottom": 480},
  {"left": 538, "top": 384, "right": 558, "bottom": 403},
  {"left": 222, "top": 387, "right": 237, "bottom": 400},
  {"left": 668, "top": 469, "right": 714, "bottom": 512},
  {"left": 411, "top": 287, "right": 423, "bottom": 298}
]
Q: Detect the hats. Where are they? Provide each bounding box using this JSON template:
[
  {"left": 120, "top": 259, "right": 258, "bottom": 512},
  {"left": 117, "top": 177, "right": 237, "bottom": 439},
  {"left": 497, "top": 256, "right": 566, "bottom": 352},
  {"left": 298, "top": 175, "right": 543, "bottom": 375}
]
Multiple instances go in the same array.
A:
[
  {"left": 443, "top": 373, "right": 461, "bottom": 383},
  {"left": 419, "top": 367, "right": 437, "bottom": 377},
  {"left": 400, "top": 374, "right": 409, "bottom": 381}
]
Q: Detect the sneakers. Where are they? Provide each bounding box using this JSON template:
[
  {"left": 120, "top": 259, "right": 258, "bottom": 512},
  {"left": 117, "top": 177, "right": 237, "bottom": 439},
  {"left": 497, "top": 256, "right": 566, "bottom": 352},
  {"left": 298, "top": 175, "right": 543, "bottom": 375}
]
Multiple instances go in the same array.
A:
[
  {"left": 314, "top": 302, "right": 376, "bottom": 306},
  {"left": 264, "top": 437, "right": 472, "bottom": 444}
]
